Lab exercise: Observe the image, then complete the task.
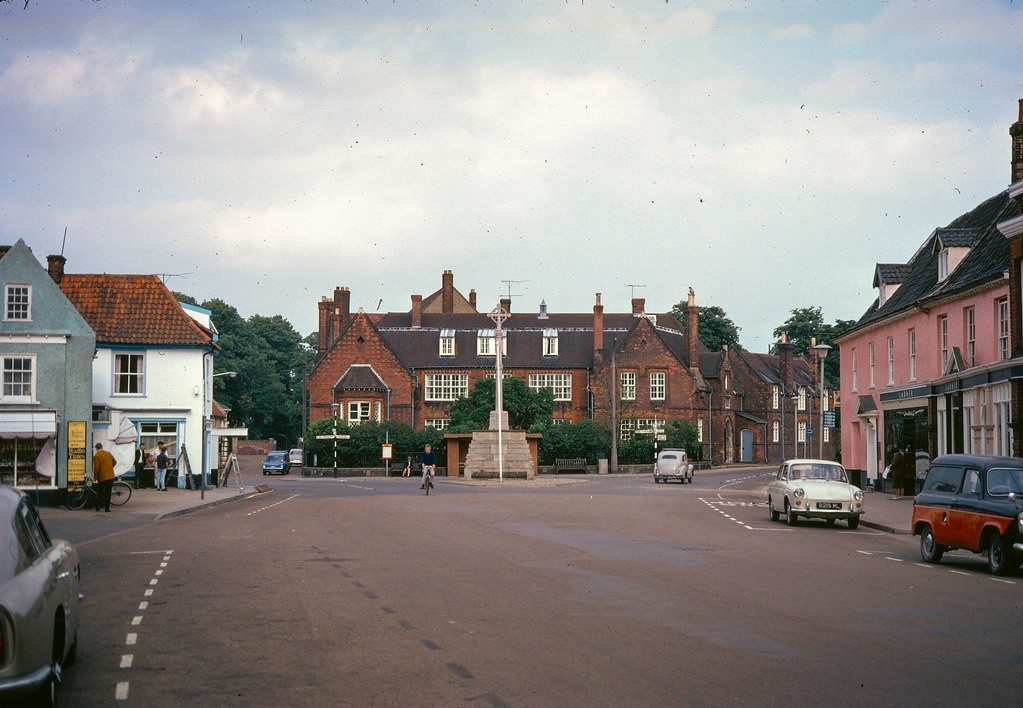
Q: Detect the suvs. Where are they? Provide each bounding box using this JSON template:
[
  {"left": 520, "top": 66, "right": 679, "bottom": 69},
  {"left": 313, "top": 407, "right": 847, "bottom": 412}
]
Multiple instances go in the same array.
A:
[{"left": 911, "top": 454, "right": 1023, "bottom": 576}]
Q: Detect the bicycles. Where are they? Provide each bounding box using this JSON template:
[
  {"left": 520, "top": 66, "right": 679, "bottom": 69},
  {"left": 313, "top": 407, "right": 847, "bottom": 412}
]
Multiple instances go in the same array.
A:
[
  {"left": 66, "top": 472, "right": 133, "bottom": 511},
  {"left": 424, "top": 466, "right": 434, "bottom": 495}
]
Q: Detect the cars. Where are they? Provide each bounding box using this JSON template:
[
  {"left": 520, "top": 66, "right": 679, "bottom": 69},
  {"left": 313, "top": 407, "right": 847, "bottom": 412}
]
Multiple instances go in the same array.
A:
[
  {"left": 767, "top": 459, "right": 865, "bottom": 529},
  {"left": 654, "top": 448, "right": 694, "bottom": 484},
  {"left": 0, "top": 485, "right": 82, "bottom": 708},
  {"left": 262, "top": 451, "right": 291, "bottom": 475},
  {"left": 289, "top": 448, "right": 303, "bottom": 466}
]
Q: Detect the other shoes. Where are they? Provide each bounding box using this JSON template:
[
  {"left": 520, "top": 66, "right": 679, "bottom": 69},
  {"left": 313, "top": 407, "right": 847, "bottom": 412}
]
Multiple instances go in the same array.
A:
[
  {"left": 891, "top": 497, "right": 902, "bottom": 501},
  {"left": 96, "top": 506, "right": 101, "bottom": 511},
  {"left": 105, "top": 509, "right": 111, "bottom": 512},
  {"left": 157, "top": 489, "right": 168, "bottom": 491},
  {"left": 402, "top": 474, "right": 410, "bottom": 478},
  {"left": 420, "top": 482, "right": 433, "bottom": 490},
  {"left": 134, "top": 485, "right": 144, "bottom": 490}
]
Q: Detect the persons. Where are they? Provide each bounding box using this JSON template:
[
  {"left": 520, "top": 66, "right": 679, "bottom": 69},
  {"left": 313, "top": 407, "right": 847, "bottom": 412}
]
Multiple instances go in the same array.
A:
[
  {"left": 150, "top": 442, "right": 168, "bottom": 489},
  {"left": 889, "top": 447, "right": 905, "bottom": 500},
  {"left": 157, "top": 446, "right": 170, "bottom": 491},
  {"left": 133, "top": 443, "right": 147, "bottom": 490},
  {"left": 420, "top": 444, "right": 437, "bottom": 489},
  {"left": 93, "top": 443, "right": 117, "bottom": 512}
]
